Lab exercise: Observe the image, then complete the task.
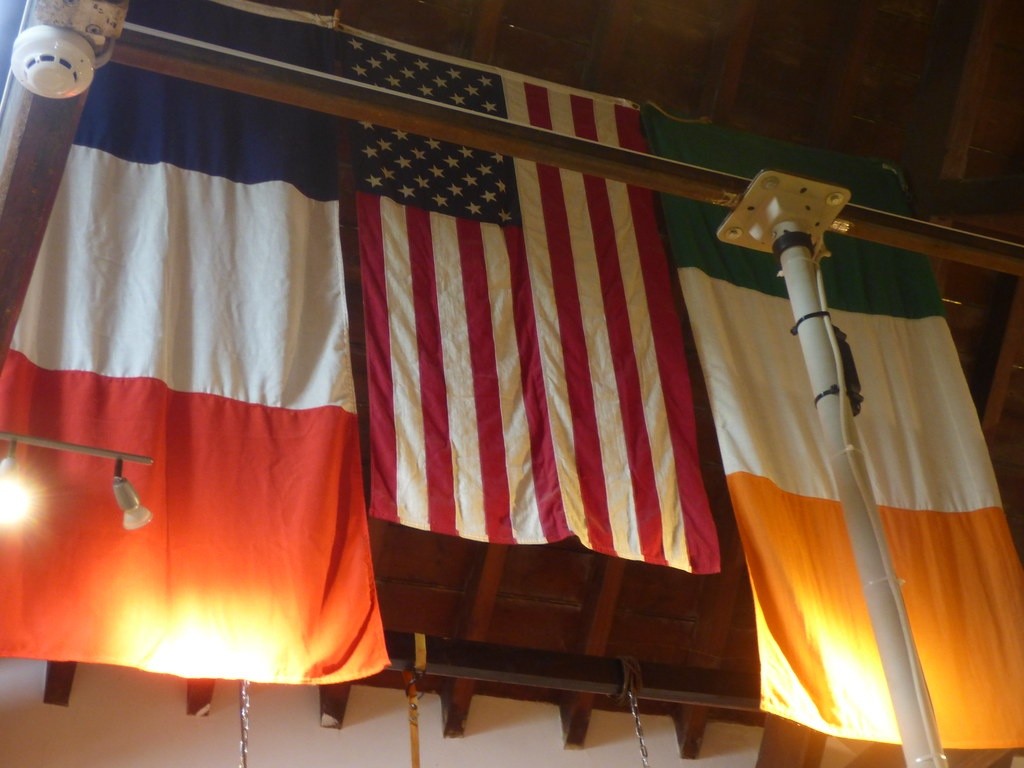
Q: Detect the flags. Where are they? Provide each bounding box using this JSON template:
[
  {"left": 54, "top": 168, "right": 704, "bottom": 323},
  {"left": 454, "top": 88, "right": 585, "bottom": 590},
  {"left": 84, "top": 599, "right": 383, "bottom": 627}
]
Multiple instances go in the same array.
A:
[
  {"left": 336, "top": 24, "right": 724, "bottom": 576},
  {"left": 0, "top": 1, "right": 392, "bottom": 685},
  {"left": 637, "top": 92, "right": 1023, "bottom": 751}
]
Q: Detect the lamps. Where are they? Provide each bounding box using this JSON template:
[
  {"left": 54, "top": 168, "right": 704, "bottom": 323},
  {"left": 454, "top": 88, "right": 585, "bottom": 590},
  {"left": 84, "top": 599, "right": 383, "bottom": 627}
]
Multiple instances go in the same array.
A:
[{"left": 112, "top": 458, "right": 153, "bottom": 531}]
